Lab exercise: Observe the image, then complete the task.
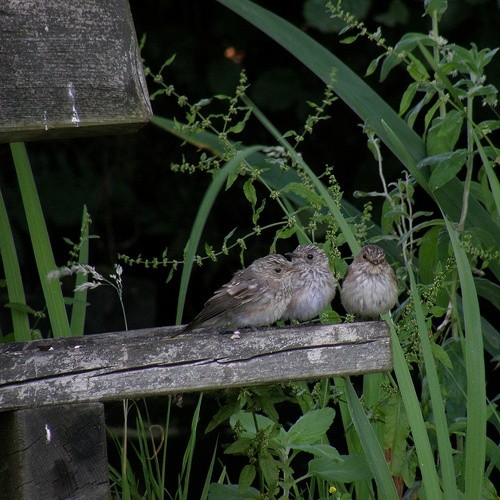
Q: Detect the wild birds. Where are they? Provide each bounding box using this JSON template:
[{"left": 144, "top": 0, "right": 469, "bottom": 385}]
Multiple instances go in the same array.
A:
[
  {"left": 340, "top": 243, "right": 399, "bottom": 320},
  {"left": 171, "top": 243, "right": 339, "bottom": 339}
]
